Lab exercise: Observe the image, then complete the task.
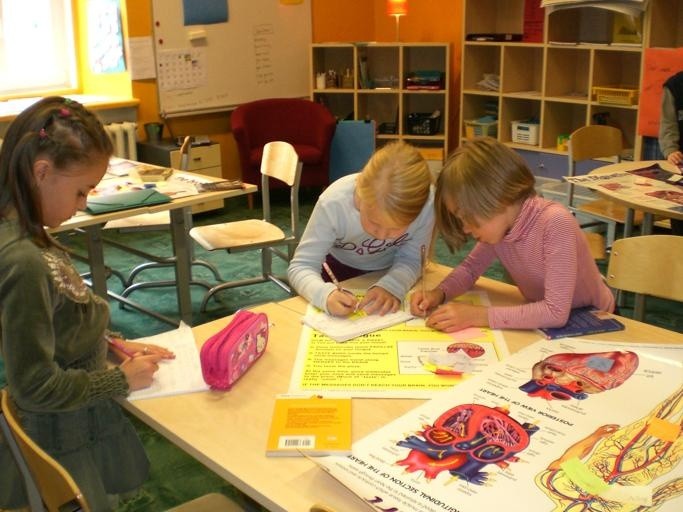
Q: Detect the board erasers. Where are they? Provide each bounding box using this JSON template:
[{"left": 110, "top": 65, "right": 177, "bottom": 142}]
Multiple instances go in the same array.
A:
[{"left": 189, "top": 30, "right": 206, "bottom": 40}]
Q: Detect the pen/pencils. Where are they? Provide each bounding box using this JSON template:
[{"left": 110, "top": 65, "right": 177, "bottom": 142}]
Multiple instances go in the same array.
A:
[
  {"left": 103, "top": 335, "right": 135, "bottom": 359},
  {"left": 421, "top": 244, "right": 427, "bottom": 322},
  {"left": 322, "top": 262, "right": 358, "bottom": 310}
]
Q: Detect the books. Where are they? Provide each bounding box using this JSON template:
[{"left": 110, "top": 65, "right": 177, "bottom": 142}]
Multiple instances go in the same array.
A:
[
  {"left": 405, "top": 70, "right": 441, "bottom": 90},
  {"left": 106, "top": 159, "right": 138, "bottom": 176},
  {"left": 534, "top": 305, "right": 624, "bottom": 341},
  {"left": 140, "top": 167, "right": 171, "bottom": 182},
  {"left": 577, "top": 40, "right": 609, "bottom": 45},
  {"left": 610, "top": 41, "right": 641, "bottom": 47},
  {"left": 263, "top": 390, "right": 352, "bottom": 458},
  {"left": 200, "top": 179, "right": 244, "bottom": 193},
  {"left": 549, "top": 40, "right": 575, "bottom": 45}
]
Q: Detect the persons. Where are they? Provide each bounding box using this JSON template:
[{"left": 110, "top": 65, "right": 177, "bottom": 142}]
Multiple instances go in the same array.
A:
[
  {"left": 0, "top": 94, "right": 176, "bottom": 512},
  {"left": 409, "top": 135, "right": 619, "bottom": 334},
  {"left": 287, "top": 140, "right": 435, "bottom": 320},
  {"left": 657, "top": 69, "right": 682, "bottom": 166}
]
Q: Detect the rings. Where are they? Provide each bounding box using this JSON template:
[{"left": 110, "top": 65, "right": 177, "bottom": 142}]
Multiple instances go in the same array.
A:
[{"left": 141, "top": 346, "right": 148, "bottom": 355}]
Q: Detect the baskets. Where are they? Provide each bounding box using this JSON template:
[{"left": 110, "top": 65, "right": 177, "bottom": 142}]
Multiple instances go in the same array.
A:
[
  {"left": 511, "top": 121, "right": 540, "bottom": 146},
  {"left": 408, "top": 113, "right": 440, "bottom": 135},
  {"left": 465, "top": 118, "right": 497, "bottom": 137},
  {"left": 523, "top": 1, "right": 544, "bottom": 41}
]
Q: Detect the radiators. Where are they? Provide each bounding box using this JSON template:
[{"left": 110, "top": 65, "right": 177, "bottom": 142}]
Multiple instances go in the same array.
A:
[{"left": 102, "top": 120, "right": 138, "bottom": 161}]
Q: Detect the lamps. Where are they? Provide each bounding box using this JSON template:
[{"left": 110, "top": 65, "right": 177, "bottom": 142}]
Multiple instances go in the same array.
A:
[{"left": 383, "top": 1, "right": 409, "bottom": 41}]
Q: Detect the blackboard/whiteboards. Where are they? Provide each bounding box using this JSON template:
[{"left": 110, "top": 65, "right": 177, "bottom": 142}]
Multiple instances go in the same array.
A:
[{"left": 150, "top": 0, "right": 314, "bottom": 121}]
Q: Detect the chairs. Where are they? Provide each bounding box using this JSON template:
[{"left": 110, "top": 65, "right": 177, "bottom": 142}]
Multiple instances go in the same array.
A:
[
  {"left": 537, "top": 190, "right": 606, "bottom": 262},
  {"left": 567, "top": 125, "right": 671, "bottom": 253},
  {"left": 188, "top": 139, "right": 304, "bottom": 313},
  {"left": 230, "top": 98, "right": 338, "bottom": 210},
  {"left": 603, "top": 235, "right": 683, "bottom": 322},
  {"left": 0, "top": 388, "right": 247, "bottom": 512},
  {"left": 118, "top": 135, "right": 223, "bottom": 310}
]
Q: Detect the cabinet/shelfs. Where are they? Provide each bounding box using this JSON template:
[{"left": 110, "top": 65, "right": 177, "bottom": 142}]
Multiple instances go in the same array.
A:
[
  {"left": 138, "top": 143, "right": 225, "bottom": 218},
  {"left": 309, "top": 40, "right": 451, "bottom": 162},
  {"left": 458, "top": 0, "right": 652, "bottom": 181}
]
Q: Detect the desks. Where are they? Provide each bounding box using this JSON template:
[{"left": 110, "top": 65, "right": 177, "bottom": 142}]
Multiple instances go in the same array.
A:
[
  {"left": 587, "top": 159, "right": 683, "bottom": 322},
  {"left": 44, "top": 155, "right": 258, "bottom": 329},
  {"left": 108, "top": 260, "right": 682, "bottom": 512}
]
